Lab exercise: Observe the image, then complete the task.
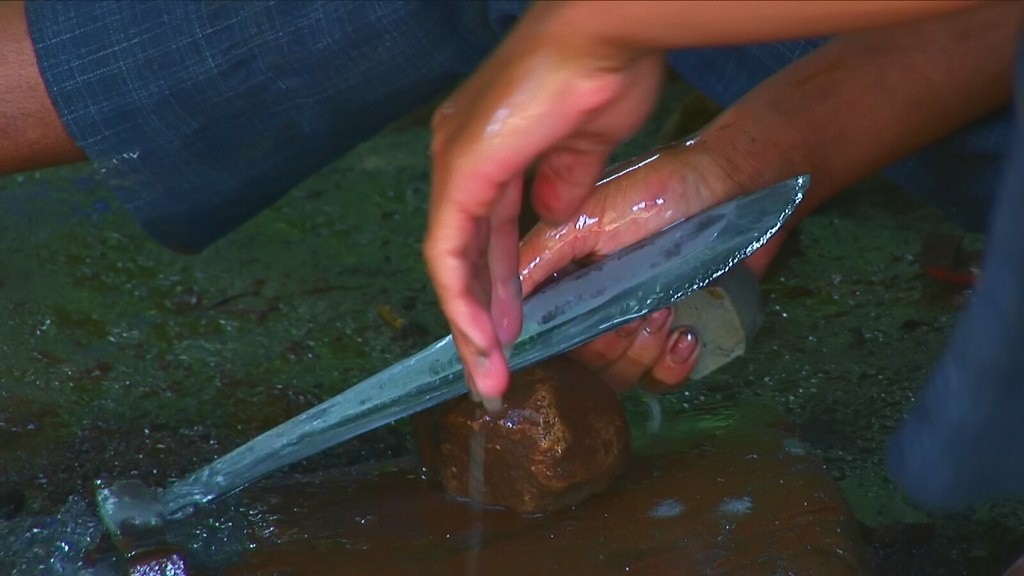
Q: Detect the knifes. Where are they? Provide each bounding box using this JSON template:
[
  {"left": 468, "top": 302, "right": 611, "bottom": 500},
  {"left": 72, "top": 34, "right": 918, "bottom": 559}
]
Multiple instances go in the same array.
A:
[{"left": 89, "top": 173, "right": 813, "bottom": 562}]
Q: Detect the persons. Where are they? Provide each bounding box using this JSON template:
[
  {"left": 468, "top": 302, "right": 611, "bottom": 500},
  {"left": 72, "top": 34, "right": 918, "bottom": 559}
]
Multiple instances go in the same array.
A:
[{"left": 0, "top": 1, "right": 1024, "bottom": 522}]
